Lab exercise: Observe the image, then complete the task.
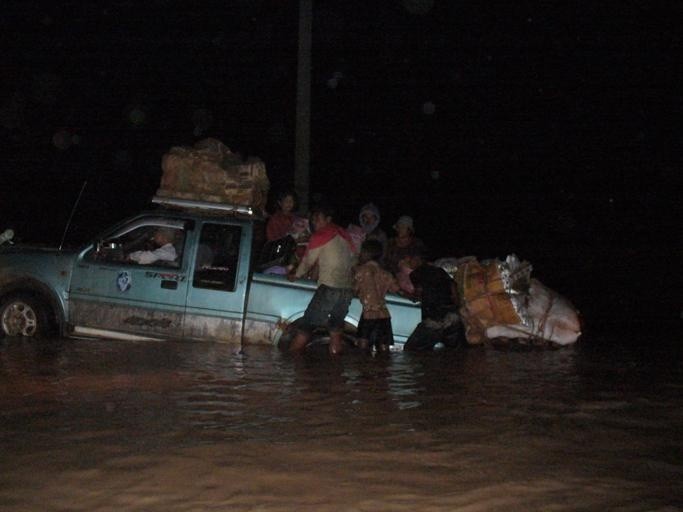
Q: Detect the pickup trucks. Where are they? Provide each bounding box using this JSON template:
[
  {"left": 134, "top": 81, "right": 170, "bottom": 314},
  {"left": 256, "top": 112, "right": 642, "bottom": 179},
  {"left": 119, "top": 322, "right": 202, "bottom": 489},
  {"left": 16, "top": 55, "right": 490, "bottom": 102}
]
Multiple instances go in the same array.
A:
[{"left": 0, "top": 218, "right": 455, "bottom": 352}]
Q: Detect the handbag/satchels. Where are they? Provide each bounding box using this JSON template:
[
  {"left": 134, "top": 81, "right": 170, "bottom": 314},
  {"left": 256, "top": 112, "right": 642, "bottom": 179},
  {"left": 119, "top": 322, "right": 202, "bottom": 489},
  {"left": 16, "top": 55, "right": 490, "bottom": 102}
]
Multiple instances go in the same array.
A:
[{"left": 255, "top": 234, "right": 297, "bottom": 272}]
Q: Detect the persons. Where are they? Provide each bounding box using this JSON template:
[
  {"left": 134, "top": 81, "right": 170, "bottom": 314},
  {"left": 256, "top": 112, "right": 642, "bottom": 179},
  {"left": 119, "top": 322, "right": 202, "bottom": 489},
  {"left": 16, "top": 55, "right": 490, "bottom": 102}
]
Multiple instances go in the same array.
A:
[
  {"left": 122, "top": 229, "right": 236, "bottom": 276},
  {"left": 266, "top": 194, "right": 463, "bottom": 354}
]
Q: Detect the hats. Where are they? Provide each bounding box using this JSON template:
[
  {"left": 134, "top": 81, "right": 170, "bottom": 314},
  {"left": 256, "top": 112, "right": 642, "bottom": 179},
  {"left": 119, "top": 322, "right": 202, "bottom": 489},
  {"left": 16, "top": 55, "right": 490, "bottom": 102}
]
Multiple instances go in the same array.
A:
[{"left": 393, "top": 215, "right": 416, "bottom": 234}]
